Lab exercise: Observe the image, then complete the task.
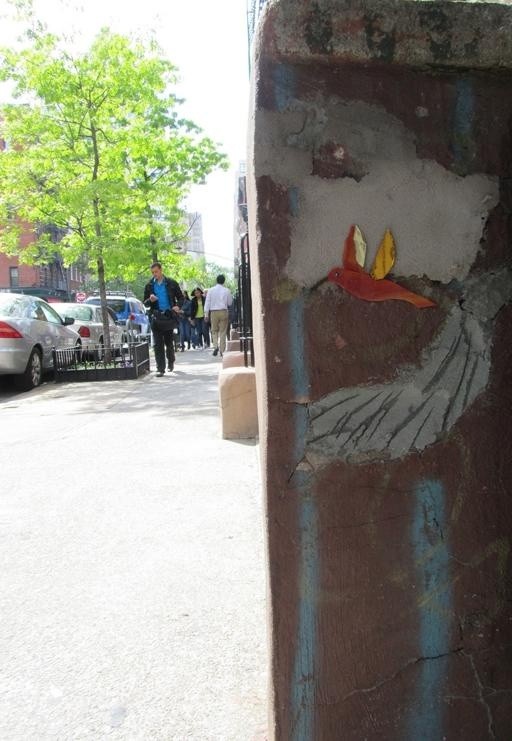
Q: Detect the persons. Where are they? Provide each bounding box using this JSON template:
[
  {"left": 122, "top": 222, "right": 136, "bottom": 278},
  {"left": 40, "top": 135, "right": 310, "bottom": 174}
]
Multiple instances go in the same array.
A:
[
  {"left": 174, "top": 287, "right": 234, "bottom": 352},
  {"left": 142, "top": 263, "right": 184, "bottom": 378},
  {"left": 203, "top": 274, "right": 234, "bottom": 356}
]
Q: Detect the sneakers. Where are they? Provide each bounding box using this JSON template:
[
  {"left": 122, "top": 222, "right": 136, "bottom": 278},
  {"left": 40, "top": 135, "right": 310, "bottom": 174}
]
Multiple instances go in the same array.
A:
[
  {"left": 168, "top": 359, "right": 173, "bottom": 372},
  {"left": 155, "top": 372, "right": 164, "bottom": 377}
]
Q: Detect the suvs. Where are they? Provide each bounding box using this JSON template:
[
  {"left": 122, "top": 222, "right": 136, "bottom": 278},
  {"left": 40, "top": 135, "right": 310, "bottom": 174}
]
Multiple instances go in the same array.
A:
[{"left": 84, "top": 290, "right": 151, "bottom": 345}]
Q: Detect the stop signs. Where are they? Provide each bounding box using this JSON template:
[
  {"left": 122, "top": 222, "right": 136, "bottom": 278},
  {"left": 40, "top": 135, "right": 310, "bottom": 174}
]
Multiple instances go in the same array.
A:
[{"left": 77, "top": 294, "right": 86, "bottom": 303}]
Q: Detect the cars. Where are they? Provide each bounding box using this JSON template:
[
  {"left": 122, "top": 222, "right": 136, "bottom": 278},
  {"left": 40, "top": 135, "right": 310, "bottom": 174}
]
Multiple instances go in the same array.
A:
[
  {"left": 0, "top": 293, "right": 83, "bottom": 392},
  {"left": 46, "top": 303, "right": 126, "bottom": 360}
]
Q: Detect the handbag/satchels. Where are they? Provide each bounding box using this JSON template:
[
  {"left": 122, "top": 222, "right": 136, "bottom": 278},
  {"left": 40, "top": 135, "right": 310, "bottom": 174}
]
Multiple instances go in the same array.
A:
[{"left": 150, "top": 308, "right": 179, "bottom": 332}]
